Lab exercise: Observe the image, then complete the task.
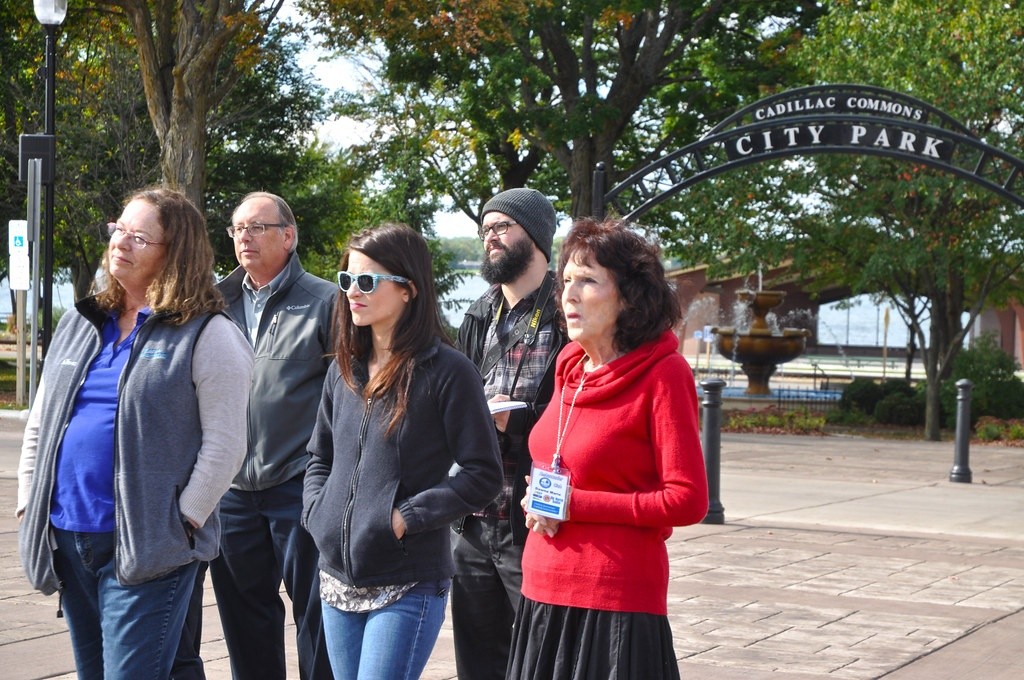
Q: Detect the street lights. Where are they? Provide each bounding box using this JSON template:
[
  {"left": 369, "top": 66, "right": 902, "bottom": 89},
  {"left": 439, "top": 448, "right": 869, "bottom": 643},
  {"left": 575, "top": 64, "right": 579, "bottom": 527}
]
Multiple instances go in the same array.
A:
[{"left": 35, "top": 0, "right": 68, "bottom": 360}]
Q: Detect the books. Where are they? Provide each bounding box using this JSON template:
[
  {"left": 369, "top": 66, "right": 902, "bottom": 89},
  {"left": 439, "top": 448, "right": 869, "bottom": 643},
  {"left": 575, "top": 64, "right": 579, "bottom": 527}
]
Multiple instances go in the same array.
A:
[{"left": 488, "top": 401, "right": 528, "bottom": 414}]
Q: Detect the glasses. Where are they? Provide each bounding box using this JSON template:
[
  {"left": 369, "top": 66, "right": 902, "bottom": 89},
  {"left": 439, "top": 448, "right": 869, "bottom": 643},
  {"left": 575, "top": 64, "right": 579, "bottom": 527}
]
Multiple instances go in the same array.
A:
[
  {"left": 107, "top": 222, "right": 165, "bottom": 249},
  {"left": 337, "top": 271, "right": 409, "bottom": 293},
  {"left": 478, "top": 220, "right": 518, "bottom": 241},
  {"left": 226, "top": 224, "right": 288, "bottom": 237}
]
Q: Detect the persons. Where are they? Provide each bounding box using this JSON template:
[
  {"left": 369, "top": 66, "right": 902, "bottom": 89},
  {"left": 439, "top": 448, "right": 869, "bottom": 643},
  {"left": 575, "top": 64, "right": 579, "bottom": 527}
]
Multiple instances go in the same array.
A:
[
  {"left": 304, "top": 222, "right": 505, "bottom": 680},
  {"left": 505, "top": 215, "right": 709, "bottom": 680},
  {"left": 209, "top": 192, "right": 340, "bottom": 680},
  {"left": 703, "top": 322, "right": 714, "bottom": 342},
  {"left": 449, "top": 189, "right": 571, "bottom": 680},
  {"left": 16, "top": 187, "right": 255, "bottom": 680}
]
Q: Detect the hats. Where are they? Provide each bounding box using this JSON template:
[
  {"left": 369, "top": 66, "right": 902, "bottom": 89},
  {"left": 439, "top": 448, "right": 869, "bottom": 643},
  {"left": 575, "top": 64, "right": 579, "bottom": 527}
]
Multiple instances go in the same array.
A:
[{"left": 480, "top": 188, "right": 556, "bottom": 263}]
